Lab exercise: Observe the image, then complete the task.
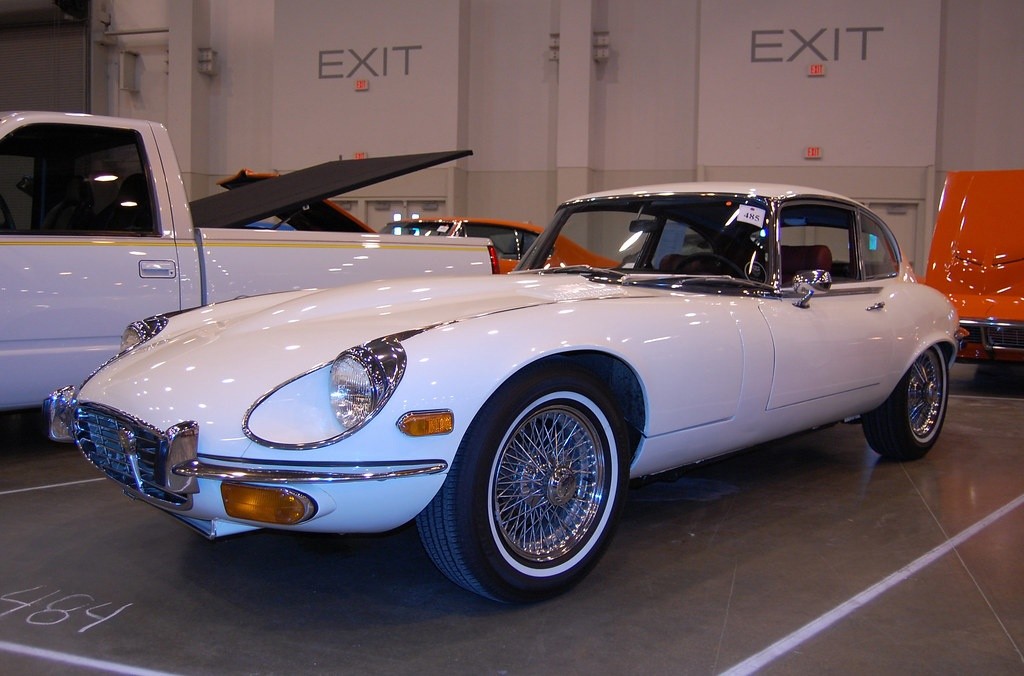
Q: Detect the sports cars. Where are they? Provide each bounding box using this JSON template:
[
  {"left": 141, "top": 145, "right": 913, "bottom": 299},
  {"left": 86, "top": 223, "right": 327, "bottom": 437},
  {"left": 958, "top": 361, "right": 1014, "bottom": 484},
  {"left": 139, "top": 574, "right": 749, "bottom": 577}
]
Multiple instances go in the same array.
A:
[
  {"left": 224, "top": 169, "right": 622, "bottom": 273},
  {"left": 40, "top": 183, "right": 960, "bottom": 605}
]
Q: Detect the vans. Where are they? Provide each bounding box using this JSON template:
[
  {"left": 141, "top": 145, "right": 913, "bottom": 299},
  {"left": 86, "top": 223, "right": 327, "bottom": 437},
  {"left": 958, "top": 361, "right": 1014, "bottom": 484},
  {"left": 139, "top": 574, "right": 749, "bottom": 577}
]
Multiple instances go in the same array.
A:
[{"left": 923, "top": 169, "right": 1023, "bottom": 368}]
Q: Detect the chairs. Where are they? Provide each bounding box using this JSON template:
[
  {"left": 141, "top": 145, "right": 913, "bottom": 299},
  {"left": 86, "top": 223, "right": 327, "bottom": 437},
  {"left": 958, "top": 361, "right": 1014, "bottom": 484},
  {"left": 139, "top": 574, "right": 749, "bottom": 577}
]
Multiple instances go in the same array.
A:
[
  {"left": 40, "top": 171, "right": 151, "bottom": 231},
  {"left": 777, "top": 244, "right": 831, "bottom": 282},
  {"left": 659, "top": 254, "right": 718, "bottom": 275}
]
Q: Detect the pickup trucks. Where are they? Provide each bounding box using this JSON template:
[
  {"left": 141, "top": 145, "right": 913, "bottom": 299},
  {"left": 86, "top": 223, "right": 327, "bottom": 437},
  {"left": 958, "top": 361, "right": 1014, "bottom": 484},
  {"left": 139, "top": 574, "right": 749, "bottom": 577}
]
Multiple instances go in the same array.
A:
[{"left": 0, "top": 108, "right": 502, "bottom": 413}]
